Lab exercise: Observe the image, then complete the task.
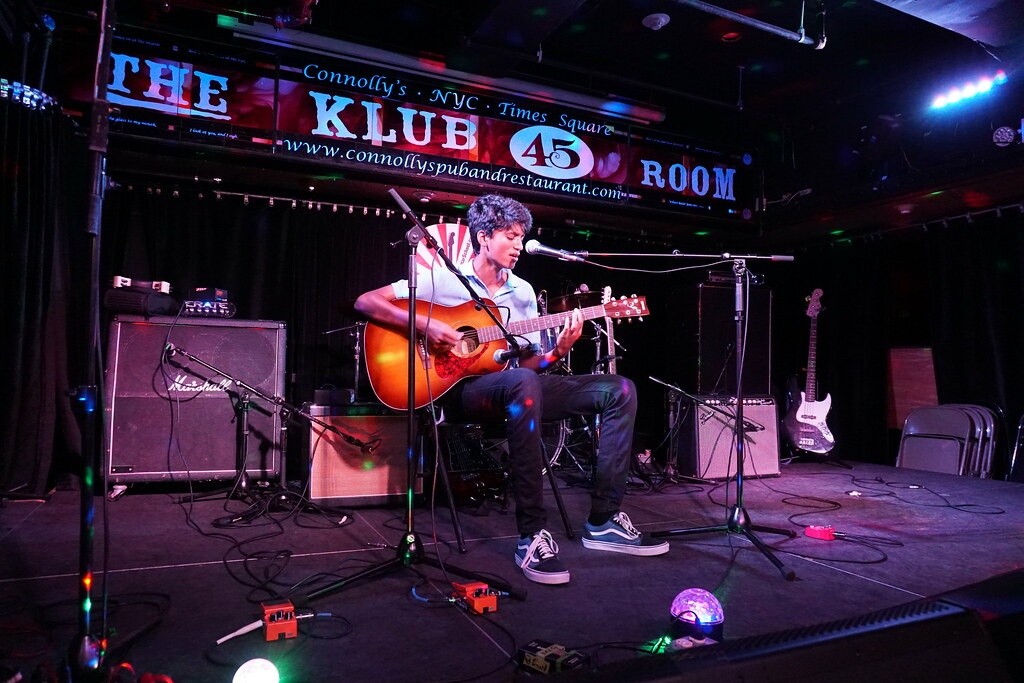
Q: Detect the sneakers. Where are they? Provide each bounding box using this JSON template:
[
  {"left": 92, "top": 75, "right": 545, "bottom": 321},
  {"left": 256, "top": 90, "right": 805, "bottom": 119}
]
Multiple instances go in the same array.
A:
[
  {"left": 581, "top": 512, "right": 669, "bottom": 556},
  {"left": 514, "top": 528, "right": 570, "bottom": 584}
]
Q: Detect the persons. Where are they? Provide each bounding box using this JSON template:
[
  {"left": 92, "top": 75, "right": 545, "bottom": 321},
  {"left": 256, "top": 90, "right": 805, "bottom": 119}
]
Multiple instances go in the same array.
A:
[{"left": 354, "top": 195, "right": 669, "bottom": 585}]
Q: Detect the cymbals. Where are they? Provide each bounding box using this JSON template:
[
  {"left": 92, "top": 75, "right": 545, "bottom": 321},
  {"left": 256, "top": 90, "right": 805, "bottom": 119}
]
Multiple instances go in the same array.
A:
[{"left": 546, "top": 287, "right": 601, "bottom": 313}]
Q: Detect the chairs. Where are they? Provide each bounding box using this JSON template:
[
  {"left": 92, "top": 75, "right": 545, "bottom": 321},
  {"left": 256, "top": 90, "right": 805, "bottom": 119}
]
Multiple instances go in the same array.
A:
[
  {"left": 895, "top": 400, "right": 1024, "bottom": 481},
  {"left": 400, "top": 405, "right": 576, "bottom": 554}
]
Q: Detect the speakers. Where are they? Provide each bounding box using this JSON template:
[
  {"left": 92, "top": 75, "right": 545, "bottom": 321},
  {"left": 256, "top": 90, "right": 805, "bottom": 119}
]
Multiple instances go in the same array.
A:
[
  {"left": 499, "top": 568, "right": 1024, "bottom": 683},
  {"left": 101, "top": 314, "right": 284, "bottom": 483},
  {"left": 676, "top": 280, "right": 781, "bottom": 482},
  {"left": 302, "top": 404, "right": 426, "bottom": 507}
]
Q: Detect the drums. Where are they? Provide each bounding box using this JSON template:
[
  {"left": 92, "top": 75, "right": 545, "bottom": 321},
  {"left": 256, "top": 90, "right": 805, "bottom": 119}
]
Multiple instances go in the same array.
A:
[{"left": 538, "top": 312, "right": 567, "bottom": 377}]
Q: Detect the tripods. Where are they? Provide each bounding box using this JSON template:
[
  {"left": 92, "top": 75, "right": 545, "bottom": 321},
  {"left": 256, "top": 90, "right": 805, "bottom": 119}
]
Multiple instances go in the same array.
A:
[
  {"left": 564, "top": 250, "right": 798, "bottom": 581},
  {"left": 164, "top": 188, "right": 529, "bottom": 608}
]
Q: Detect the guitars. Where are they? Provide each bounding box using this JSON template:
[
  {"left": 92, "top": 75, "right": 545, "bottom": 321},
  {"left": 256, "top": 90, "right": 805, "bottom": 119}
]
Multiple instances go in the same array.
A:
[
  {"left": 784, "top": 288, "right": 838, "bottom": 456},
  {"left": 599, "top": 286, "right": 618, "bottom": 375},
  {"left": 363, "top": 291, "right": 652, "bottom": 414}
]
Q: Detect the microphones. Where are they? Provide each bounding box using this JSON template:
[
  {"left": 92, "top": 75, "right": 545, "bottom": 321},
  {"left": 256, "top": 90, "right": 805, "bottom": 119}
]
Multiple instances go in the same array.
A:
[
  {"left": 525, "top": 239, "right": 585, "bottom": 262},
  {"left": 579, "top": 284, "right": 589, "bottom": 292},
  {"left": 166, "top": 342, "right": 176, "bottom": 357},
  {"left": 537, "top": 290, "right": 545, "bottom": 302},
  {"left": 493, "top": 344, "right": 533, "bottom": 364},
  {"left": 744, "top": 427, "right": 764, "bottom": 432}
]
